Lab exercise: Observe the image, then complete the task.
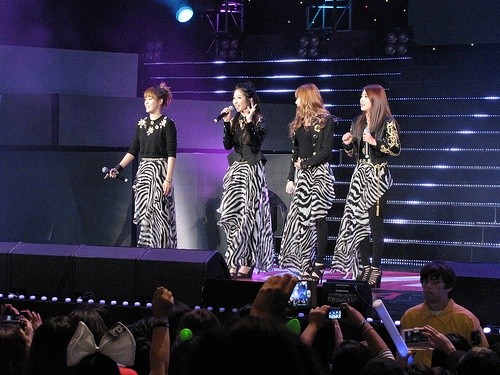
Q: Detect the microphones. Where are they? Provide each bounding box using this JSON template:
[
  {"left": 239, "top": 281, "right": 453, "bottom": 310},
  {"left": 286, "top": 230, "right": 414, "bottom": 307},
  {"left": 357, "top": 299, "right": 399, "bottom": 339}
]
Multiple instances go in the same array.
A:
[
  {"left": 287, "top": 187, "right": 293, "bottom": 192},
  {"left": 102, "top": 167, "right": 128, "bottom": 183},
  {"left": 213, "top": 106, "right": 234, "bottom": 123},
  {"left": 364, "top": 127, "right": 371, "bottom": 159}
]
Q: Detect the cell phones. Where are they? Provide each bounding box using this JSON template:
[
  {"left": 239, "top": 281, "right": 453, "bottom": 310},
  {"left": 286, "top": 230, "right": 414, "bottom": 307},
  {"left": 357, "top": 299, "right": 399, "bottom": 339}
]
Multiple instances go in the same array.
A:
[
  {"left": 401, "top": 327, "right": 432, "bottom": 345},
  {"left": 326, "top": 307, "right": 347, "bottom": 319},
  {"left": 1, "top": 314, "right": 23, "bottom": 323},
  {"left": 286, "top": 281, "right": 317, "bottom": 308}
]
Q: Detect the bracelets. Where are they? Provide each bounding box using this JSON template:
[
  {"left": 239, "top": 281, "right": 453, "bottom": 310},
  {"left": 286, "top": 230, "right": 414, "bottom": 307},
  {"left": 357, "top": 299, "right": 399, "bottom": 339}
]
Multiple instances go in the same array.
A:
[{"left": 164, "top": 178, "right": 173, "bottom": 183}]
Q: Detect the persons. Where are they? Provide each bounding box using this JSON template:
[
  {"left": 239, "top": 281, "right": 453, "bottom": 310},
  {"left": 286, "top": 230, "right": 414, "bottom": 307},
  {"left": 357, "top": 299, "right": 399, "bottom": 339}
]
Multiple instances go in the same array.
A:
[
  {"left": 103, "top": 81, "right": 178, "bottom": 249},
  {"left": 276, "top": 83, "right": 337, "bottom": 284},
  {"left": 329, "top": 82, "right": 402, "bottom": 288},
  {"left": 1, "top": 261, "right": 499, "bottom": 375},
  {"left": 217, "top": 80, "right": 277, "bottom": 280}
]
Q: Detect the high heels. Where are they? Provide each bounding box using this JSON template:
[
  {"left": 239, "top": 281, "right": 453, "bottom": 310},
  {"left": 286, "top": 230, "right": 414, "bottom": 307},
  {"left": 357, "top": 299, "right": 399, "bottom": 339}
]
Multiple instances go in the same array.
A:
[
  {"left": 301, "top": 263, "right": 325, "bottom": 284},
  {"left": 229, "top": 264, "right": 256, "bottom": 278},
  {"left": 361, "top": 267, "right": 381, "bottom": 289}
]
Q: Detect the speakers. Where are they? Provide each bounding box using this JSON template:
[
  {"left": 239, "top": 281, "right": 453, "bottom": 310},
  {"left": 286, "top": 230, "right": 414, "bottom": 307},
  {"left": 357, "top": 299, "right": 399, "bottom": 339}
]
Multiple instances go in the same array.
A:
[
  {"left": 323, "top": 278, "right": 373, "bottom": 310},
  {"left": 1, "top": 242, "right": 235, "bottom": 297},
  {"left": 447, "top": 277, "right": 500, "bottom": 326}
]
